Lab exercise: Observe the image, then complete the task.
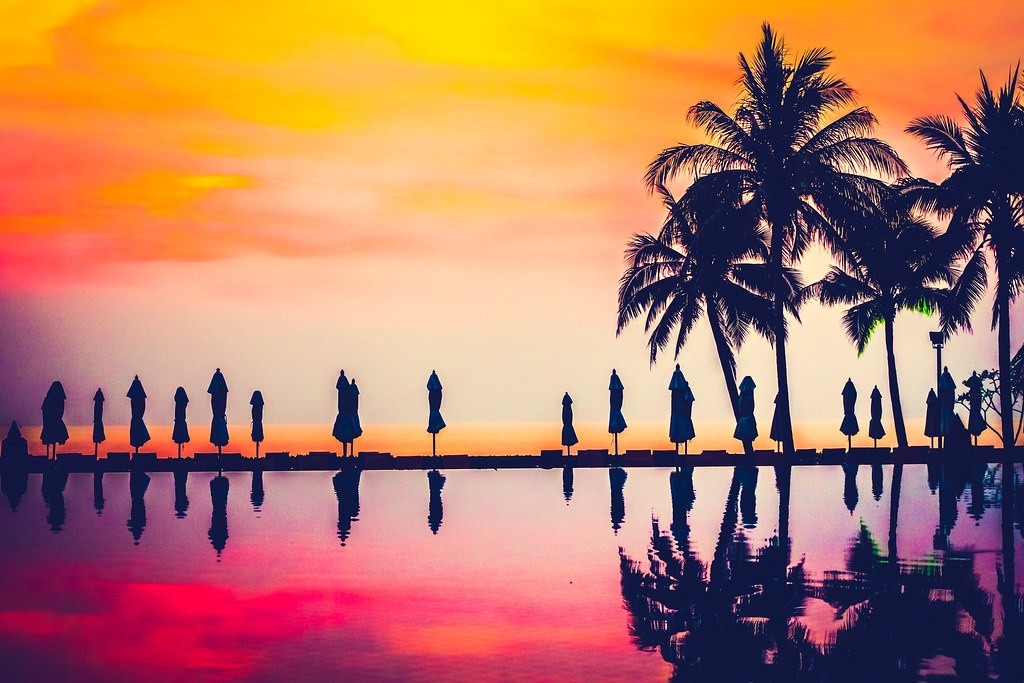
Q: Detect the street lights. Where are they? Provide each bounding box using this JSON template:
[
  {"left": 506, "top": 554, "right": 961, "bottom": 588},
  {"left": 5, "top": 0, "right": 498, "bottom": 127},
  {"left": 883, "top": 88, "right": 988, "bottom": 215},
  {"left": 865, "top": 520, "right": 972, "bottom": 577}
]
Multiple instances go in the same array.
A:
[{"left": 929, "top": 331, "right": 944, "bottom": 449}]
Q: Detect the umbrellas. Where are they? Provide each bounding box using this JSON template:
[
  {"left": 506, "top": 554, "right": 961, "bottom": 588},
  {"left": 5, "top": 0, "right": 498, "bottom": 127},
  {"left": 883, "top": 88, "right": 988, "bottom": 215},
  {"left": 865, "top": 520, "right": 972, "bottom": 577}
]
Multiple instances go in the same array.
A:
[
  {"left": 39, "top": 368, "right": 265, "bottom": 460},
  {"left": 609, "top": 467, "right": 628, "bottom": 536},
  {"left": 426, "top": 370, "right": 446, "bottom": 456},
  {"left": 426, "top": 469, "right": 446, "bottom": 535},
  {"left": 41, "top": 472, "right": 265, "bottom": 563},
  {"left": 670, "top": 464, "right": 696, "bottom": 556},
  {"left": 332, "top": 369, "right": 363, "bottom": 456},
  {"left": 608, "top": 369, "right": 627, "bottom": 455},
  {"left": 733, "top": 376, "right": 758, "bottom": 442},
  {"left": 331, "top": 469, "right": 362, "bottom": 546},
  {"left": 738, "top": 464, "right": 988, "bottom": 530},
  {"left": 668, "top": 363, "right": 695, "bottom": 455},
  {"left": 770, "top": 365, "right": 988, "bottom": 453},
  {"left": 562, "top": 467, "right": 574, "bottom": 506},
  {"left": 562, "top": 392, "right": 578, "bottom": 455}
]
